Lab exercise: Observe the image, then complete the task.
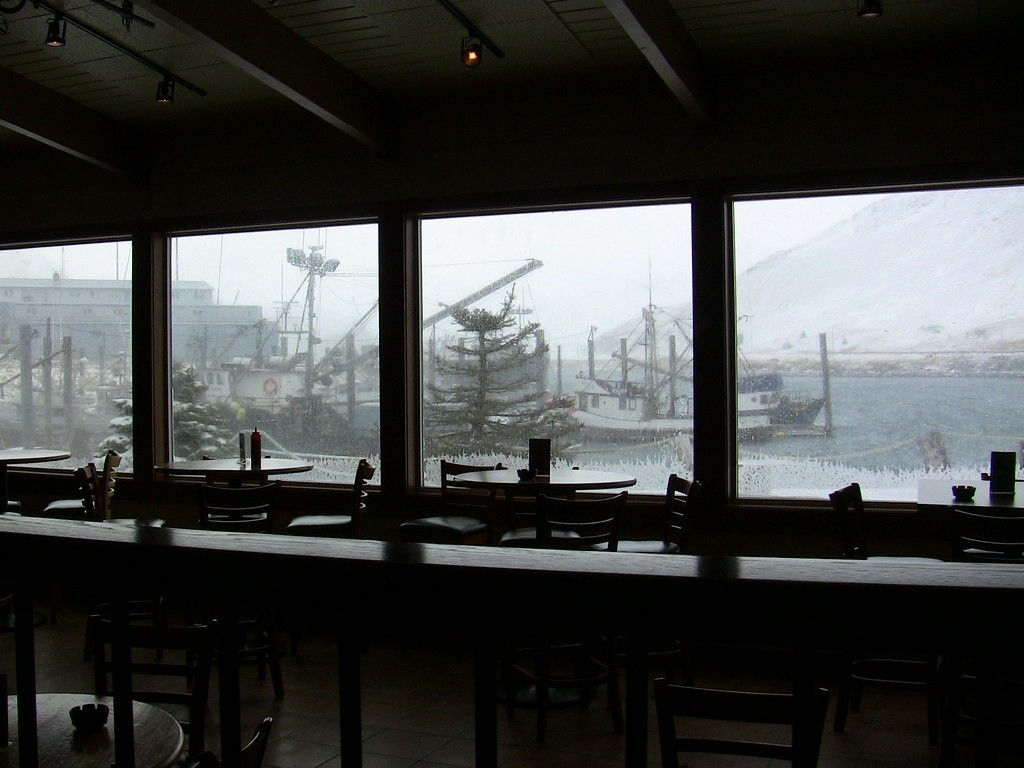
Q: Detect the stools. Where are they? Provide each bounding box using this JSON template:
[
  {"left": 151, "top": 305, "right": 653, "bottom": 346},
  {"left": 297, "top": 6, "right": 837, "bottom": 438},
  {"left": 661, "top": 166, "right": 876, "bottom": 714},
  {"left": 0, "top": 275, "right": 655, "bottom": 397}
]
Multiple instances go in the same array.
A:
[
  {"left": 43, "top": 451, "right": 120, "bottom": 624},
  {"left": 81, "top": 463, "right": 166, "bottom": 531},
  {"left": 507, "top": 492, "right": 628, "bottom": 741},
  {"left": 286, "top": 459, "right": 368, "bottom": 657},
  {"left": 594, "top": 475, "right": 700, "bottom": 687},
  {"left": 401, "top": 460, "right": 501, "bottom": 656},
  {"left": 189, "top": 480, "right": 284, "bottom": 702},
  {"left": 649, "top": 677, "right": 828, "bottom": 767},
  {"left": 87, "top": 613, "right": 216, "bottom": 761}
]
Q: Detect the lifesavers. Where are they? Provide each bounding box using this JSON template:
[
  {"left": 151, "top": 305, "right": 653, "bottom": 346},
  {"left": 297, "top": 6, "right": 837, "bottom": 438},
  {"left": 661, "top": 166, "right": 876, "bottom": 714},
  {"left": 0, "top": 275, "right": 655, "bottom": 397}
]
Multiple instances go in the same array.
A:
[{"left": 263, "top": 377, "right": 278, "bottom": 395}]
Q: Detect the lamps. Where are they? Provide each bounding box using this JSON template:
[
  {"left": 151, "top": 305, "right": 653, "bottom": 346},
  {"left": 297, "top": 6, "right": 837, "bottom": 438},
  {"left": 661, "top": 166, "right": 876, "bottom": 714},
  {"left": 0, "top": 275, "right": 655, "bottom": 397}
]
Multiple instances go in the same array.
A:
[
  {"left": 857, "top": 0, "right": 882, "bottom": 17},
  {"left": 458, "top": 32, "right": 485, "bottom": 67},
  {"left": 154, "top": 79, "right": 176, "bottom": 103},
  {"left": 45, "top": 16, "right": 68, "bottom": 48}
]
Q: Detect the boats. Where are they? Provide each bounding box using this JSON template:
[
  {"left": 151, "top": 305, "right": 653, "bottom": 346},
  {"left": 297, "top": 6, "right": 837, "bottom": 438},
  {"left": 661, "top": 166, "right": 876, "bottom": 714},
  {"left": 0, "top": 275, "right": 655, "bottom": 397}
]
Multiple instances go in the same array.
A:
[{"left": 189, "top": 243, "right": 828, "bottom": 471}]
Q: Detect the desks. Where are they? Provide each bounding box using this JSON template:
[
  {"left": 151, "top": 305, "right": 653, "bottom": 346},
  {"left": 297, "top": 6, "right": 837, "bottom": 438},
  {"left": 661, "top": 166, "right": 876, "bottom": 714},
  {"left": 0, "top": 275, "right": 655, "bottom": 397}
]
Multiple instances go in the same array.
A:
[
  {"left": 0, "top": 457, "right": 70, "bottom": 631},
  {"left": 448, "top": 468, "right": 635, "bottom": 546},
  {"left": 155, "top": 462, "right": 311, "bottom": 638},
  {"left": 1, "top": 692, "right": 185, "bottom": 768}
]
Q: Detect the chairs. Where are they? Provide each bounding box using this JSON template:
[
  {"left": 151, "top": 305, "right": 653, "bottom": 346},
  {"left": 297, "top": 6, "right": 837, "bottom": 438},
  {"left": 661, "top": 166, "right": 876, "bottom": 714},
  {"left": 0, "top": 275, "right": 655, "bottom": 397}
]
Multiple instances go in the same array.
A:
[
  {"left": 831, "top": 483, "right": 945, "bottom": 746},
  {"left": 953, "top": 510, "right": 1024, "bottom": 745}
]
